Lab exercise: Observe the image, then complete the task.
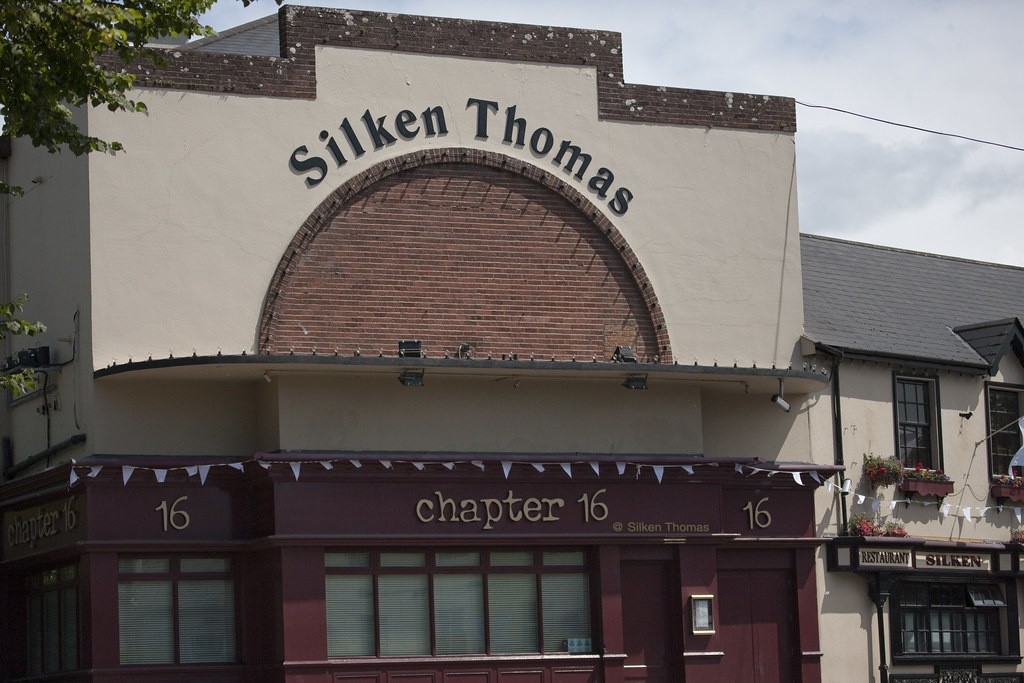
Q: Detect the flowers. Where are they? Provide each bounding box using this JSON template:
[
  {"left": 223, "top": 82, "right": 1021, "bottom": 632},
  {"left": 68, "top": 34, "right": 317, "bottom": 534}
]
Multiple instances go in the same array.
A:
[
  {"left": 902, "top": 462, "right": 946, "bottom": 480},
  {"left": 848, "top": 518, "right": 882, "bottom": 536},
  {"left": 1010, "top": 530, "right": 1024, "bottom": 543},
  {"left": 861, "top": 452, "right": 906, "bottom": 489},
  {"left": 886, "top": 522, "right": 906, "bottom": 537},
  {"left": 996, "top": 476, "right": 1024, "bottom": 488}
]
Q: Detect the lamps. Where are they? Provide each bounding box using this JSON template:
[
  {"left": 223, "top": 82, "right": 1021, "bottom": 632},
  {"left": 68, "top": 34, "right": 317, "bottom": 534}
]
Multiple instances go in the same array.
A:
[
  {"left": 399, "top": 371, "right": 425, "bottom": 387},
  {"left": 398, "top": 340, "right": 423, "bottom": 358},
  {"left": 610, "top": 345, "right": 637, "bottom": 362},
  {"left": 621, "top": 374, "right": 649, "bottom": 390}
]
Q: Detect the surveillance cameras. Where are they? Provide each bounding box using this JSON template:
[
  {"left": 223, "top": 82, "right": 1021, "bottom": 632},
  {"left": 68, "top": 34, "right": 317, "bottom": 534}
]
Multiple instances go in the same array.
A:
[
  {"left": 771, "top": 394, "right": 791, "bottom": 412},
  {"left": 841, "top": 478, "right": 852, "bottom": 495}
]
